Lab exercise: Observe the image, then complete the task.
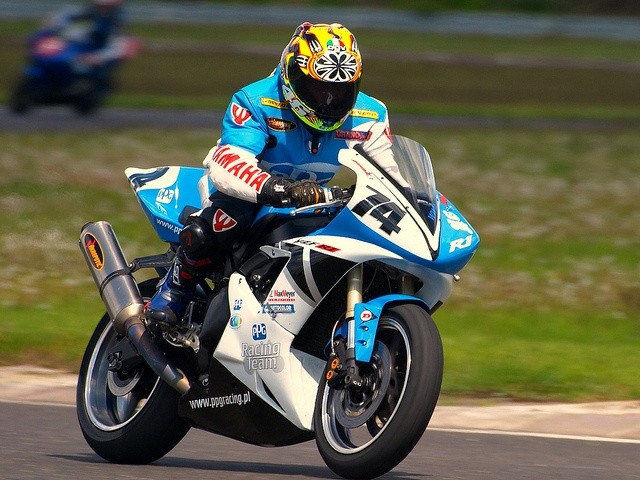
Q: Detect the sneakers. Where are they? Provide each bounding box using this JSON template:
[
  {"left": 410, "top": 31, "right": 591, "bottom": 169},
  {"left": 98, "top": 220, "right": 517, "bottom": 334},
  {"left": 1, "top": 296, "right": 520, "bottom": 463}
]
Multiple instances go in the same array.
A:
[{"left": 142, "top": 246, "right": 197, "bottom": 328}]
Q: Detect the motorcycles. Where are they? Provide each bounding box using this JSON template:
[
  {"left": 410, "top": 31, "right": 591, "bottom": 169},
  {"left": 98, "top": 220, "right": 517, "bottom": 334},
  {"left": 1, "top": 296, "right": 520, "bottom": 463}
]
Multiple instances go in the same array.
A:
[
  {"left": 11, "top": 26, "right": 144, "bottom": 116},
  {"left": 77, "top": 135, "right": 480, "bottom": 479}
]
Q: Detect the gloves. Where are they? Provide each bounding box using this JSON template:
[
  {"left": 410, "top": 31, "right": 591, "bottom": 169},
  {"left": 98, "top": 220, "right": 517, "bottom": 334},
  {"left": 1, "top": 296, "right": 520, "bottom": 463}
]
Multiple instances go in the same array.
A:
[{"left": 260, "top": 177, "right": 325, "bottom": 209}]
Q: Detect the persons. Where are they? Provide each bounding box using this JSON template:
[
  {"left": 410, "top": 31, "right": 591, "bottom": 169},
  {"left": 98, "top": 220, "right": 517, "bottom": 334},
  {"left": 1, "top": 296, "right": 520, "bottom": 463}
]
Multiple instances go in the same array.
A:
[
  {"left": 66, "top": 1, "right": 123, "bottom": 56},
  {"left": 143, "top": 21, "right": 412, "bottom": 326}
]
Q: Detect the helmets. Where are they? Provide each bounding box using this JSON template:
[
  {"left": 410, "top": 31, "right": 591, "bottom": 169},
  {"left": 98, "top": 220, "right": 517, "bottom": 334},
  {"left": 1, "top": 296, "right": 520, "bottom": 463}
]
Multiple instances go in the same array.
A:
[{"left": 279, "top": 22, "right": 364, "bottom": 134}]
